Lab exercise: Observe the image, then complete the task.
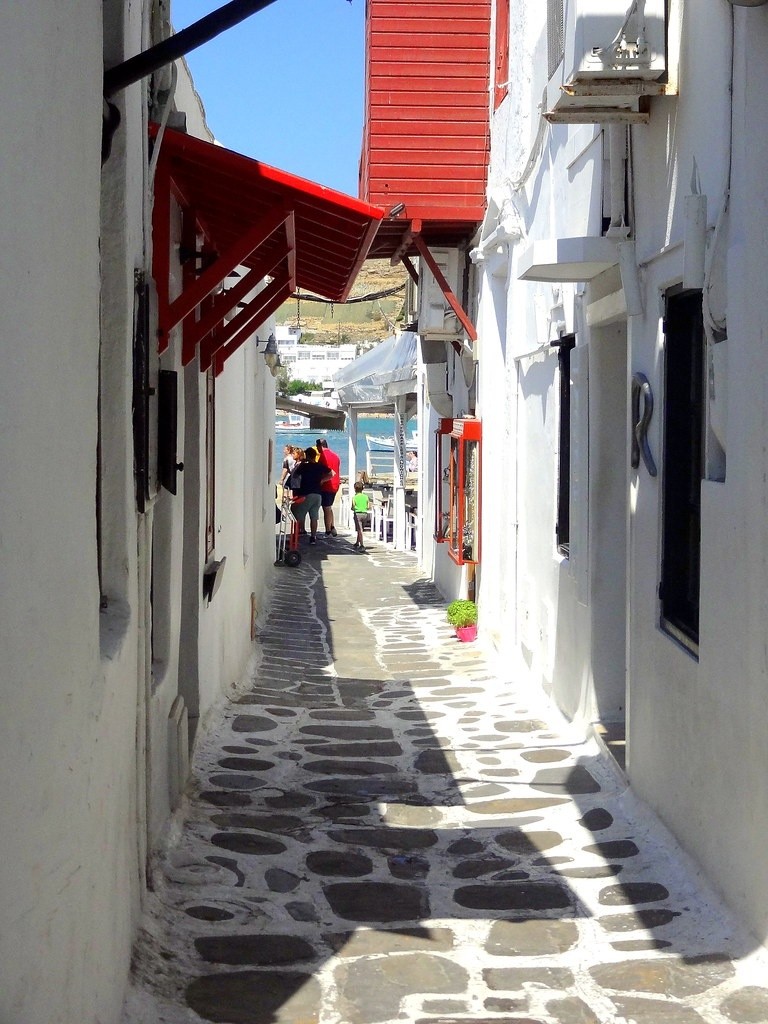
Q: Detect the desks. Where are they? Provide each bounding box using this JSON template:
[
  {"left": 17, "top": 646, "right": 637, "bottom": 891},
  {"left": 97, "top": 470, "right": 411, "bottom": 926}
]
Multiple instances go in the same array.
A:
[{"left": 375, "top": 497, "right": 393, "bottom": 538}]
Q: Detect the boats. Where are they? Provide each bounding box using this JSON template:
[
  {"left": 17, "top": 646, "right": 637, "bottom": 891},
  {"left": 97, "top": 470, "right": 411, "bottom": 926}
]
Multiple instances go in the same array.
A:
[
  {"left": 275, "top": 413, "right": 328, "bottom": 435},
  {"left": 365, "top": 428, "right": 417, "bottom": 450}
]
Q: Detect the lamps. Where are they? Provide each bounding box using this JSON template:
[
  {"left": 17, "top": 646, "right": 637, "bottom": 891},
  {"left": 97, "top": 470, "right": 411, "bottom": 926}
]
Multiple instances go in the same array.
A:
[
  {"left": 266, "top": 354, "right": 285, "bottom": 377},
  {"left": 179, "top": 244, "right": 240, "bottom": 294},
  {"left": 256, "top": 333, "right": 278, "bottom": 369},
  {"left": 223, "top": 286, "right": 248, "bottom": 321}
]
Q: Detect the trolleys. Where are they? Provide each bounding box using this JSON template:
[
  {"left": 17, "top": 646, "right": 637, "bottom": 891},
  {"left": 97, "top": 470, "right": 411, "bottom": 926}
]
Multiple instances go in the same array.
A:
[{"left": 274, "top": 483, "right": 306, "bottom": 568}]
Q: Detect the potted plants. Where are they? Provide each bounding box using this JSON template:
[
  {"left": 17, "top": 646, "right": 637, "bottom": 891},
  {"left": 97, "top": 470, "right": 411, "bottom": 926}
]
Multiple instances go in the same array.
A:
[{"left": 446, "top": 598, "right": 479, "bottom": 643}]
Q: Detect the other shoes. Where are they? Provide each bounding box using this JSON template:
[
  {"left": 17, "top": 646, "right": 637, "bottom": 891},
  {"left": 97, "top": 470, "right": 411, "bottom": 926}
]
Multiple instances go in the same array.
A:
[
  {"left": 353, "top": 543, "right": 366, "bottom": 552},
  {"left": 309, "top": 535, "right": 316, "bottom": 544},
  {"left": 299, "top": 531, "right": 307, "bottom": 535}
]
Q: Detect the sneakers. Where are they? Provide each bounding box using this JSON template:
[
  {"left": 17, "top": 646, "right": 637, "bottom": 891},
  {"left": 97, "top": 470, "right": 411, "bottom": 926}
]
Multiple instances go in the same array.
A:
[{"left": 323, "top": 526, "right": 337, "bottom": 538}]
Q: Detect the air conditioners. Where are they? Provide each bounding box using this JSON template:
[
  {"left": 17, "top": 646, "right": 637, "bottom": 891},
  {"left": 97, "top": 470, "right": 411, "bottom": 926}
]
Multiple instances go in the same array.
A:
[
  {"left": 418, "top": 246, "right": 466, "bottom": 341},
  {"left": 409, "top": 275, "right": 417, "bottom": 315},
  {"left": 541, "top": 0, "right": 682, "bottom": 124},
  {"left": 405, "top": 279, "right": 409, "bottom": 332}
]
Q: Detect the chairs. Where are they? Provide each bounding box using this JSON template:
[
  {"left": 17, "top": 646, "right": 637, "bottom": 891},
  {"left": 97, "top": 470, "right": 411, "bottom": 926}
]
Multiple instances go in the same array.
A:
[{"left": 339, "top": 483, "right": 417, "bottom": 550}]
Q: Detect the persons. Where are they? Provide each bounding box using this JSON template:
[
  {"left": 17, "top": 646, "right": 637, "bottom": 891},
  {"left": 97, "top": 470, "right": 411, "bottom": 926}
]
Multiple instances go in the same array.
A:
[
  {"left": 409, "top": 451, "right": 418, "bottom": 472},
  {"left": 279, "top": 444, "right": 296, "bottom": 500},
  {"left": 316, "top": 438, "right": 341, "bottom": 540},
  {"left": 291, "top": 448, "right": 337, "bottom": 545},
  {"left": 351, "top": 481, "right": 370, "bottom": 552},
  {"left": 289, "top": 448, "right": 304, "bottom": 474}
]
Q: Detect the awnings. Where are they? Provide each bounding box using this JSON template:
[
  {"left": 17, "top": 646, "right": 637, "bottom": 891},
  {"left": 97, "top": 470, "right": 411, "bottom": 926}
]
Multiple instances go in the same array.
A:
[
  {"left": 148, "top": 121, "right": 386, "bottom": 307},
  {"left": 276, "top": 396, "right": 346, "bottom": 431}
]
